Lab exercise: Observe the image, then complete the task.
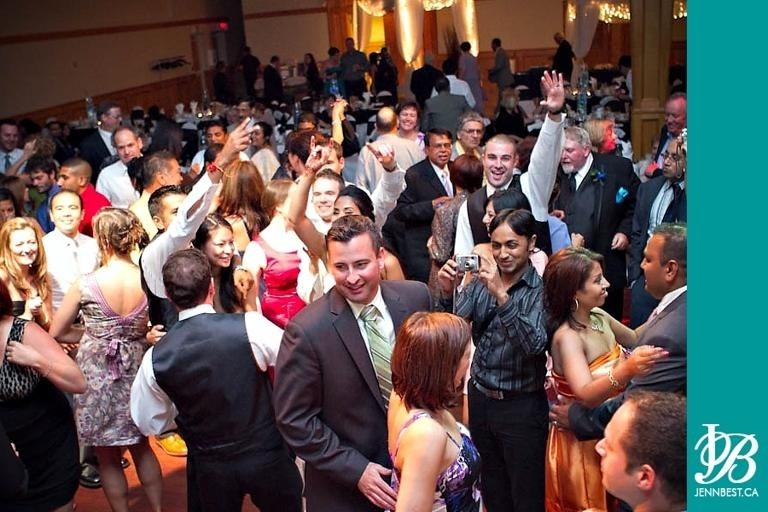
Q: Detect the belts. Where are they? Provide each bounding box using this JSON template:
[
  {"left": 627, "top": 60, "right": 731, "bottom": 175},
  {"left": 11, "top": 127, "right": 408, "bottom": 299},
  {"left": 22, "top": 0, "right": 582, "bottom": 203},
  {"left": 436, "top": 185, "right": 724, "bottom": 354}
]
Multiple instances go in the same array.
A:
[{"left": 473, "top": 380, "right": 517, "bottom": 400}]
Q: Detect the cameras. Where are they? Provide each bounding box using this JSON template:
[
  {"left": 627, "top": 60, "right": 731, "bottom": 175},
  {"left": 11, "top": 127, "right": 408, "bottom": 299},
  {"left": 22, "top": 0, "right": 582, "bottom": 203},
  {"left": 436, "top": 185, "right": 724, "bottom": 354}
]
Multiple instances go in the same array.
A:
[{"left": 453, "top": 254, "right": 481, "bottom": 272}]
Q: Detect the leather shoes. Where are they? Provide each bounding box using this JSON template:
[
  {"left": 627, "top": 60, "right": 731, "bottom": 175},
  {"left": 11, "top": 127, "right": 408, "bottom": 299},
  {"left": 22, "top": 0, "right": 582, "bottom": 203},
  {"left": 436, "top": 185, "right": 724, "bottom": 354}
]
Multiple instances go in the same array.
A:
[
  {"left": 120, "top": 456, "right": 129, "bottom": 469},
  {"left": 79, "top": 464, "right": 102, "bottom": 487},
  {"left": 155, "top": 434, "right": 188, "bottom": 456}
]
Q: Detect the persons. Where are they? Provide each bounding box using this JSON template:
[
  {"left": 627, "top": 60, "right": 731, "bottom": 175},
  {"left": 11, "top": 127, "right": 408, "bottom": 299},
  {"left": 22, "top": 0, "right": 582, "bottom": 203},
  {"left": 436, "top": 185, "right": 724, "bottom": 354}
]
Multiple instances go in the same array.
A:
[{"left": 1, "top": 31, "right": 686, "bottom": 512}]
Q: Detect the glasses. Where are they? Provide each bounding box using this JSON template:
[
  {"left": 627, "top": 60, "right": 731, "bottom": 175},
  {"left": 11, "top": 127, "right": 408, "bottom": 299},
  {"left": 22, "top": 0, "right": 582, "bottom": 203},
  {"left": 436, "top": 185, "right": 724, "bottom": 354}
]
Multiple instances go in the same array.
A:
[
  {"left": 460, "top": 127, "right": 483, "bottom": 135},
  {"left": 660, "top": 151, "right": 679, "bottom": 162}
]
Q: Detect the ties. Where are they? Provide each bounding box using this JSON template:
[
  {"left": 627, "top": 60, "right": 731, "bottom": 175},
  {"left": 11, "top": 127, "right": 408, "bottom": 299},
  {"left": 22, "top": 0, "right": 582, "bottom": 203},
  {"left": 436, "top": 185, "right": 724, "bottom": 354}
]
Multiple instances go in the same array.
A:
[
  {"left": 568, "top": 171, "right": 578, "bottom": 193},
  {"left": 442, "top": 171, "right": 452, "bottom": 196},
  {"left": 360, "top": 304, "right": 393, "bottom": 408},
  {"left": 671, "top": 183, "right": 680, "bottom": 221}
]
